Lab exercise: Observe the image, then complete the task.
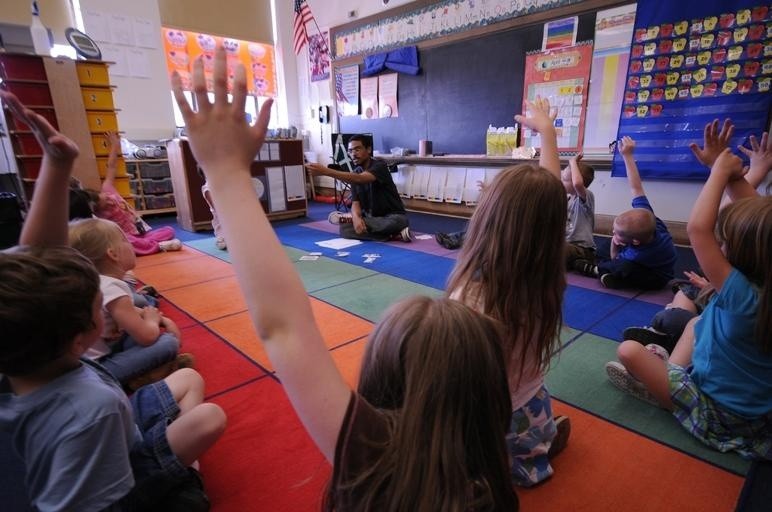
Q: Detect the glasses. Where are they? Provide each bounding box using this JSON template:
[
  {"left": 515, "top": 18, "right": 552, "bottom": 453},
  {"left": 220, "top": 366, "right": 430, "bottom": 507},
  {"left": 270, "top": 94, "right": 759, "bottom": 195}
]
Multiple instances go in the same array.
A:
[{"left": 348, "top": 149, "right": 361, "bottom": 156}]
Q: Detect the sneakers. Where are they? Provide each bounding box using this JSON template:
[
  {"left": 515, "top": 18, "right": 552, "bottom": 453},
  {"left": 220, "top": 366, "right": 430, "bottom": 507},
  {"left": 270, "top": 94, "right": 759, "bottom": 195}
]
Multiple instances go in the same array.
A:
[
  {"left": 328, "top": 211, "right": 354, "bottom": 225},
  {"left": 573, "top": 259, "right": 597, "bottom": 278},
  {"left": 644, "top": 343, "right": 669, "bottom": 361},
  {"left": 548, "top": 416, "right": 570, "bottom": 459},
  {"left": 158, "top": 239, "right": 181, "bottom": 252},
  {"left": 394, "top": 227, "right": 413, "bottom": 242},
  {"left": 605, "top": 361, "right": 665, "bottom": 410},
  {"left": 601, "top": 273, "right": 615, "bottom": 288},
  {"left": 137, "top": 285, "right": 158, "bottom": 298},
  {"left": 623, "top": 327, "right": 673, "bottom": 354},
  {"left": 174, "top": 466, "right": 211, "bottom": 511},
  {"left": 129, "top": 353, "right": 194, "bottom": 392},
  {"left": 435, "top": 231, "right": 454, "bottom": 249}
]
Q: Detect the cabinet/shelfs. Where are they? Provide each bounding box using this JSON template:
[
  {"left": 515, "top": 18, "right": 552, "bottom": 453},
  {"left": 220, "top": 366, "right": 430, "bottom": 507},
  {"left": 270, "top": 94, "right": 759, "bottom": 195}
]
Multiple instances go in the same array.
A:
[
  {"left": 0, "top": 52, "right": 133, "bottom": 214},
  {"left": 123, "top": 158, "right": 176, "bottom": 216}
]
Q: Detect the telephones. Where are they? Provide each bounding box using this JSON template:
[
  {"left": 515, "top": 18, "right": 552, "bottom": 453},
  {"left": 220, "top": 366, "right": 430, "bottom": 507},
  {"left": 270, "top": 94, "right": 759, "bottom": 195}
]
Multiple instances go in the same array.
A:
[{"left": 318, "top": 105, "right": 329, "bottom": 125}]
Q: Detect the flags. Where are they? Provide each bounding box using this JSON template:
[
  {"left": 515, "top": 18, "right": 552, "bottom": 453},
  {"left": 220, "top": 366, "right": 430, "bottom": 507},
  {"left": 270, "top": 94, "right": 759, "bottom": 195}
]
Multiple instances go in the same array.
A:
[{"left": 290, "top": 0, "right": 314, "bottom": 58}]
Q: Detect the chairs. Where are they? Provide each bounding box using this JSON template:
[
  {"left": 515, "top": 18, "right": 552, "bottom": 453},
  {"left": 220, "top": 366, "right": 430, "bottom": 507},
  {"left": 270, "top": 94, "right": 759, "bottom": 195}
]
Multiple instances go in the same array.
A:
[{"left": 326, "top": 131, "right": 373, "bottom": 212}]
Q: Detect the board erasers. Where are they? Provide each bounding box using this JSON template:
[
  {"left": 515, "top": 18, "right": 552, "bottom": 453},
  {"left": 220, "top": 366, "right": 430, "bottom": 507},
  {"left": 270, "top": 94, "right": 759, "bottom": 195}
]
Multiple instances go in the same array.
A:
[{"left": 432, "top": 152, "right": 448, "bottom": 157}]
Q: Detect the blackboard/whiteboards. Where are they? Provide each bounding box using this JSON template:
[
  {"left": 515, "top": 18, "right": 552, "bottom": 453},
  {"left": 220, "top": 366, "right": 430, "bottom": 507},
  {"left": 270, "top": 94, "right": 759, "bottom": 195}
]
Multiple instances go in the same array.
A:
[{"left": 331, "top": 0, "right": 636, "bottom": 170}]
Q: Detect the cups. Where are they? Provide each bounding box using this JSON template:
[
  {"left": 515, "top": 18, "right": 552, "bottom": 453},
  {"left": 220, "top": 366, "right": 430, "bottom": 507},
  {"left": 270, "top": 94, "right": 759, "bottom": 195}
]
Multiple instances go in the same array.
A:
[{"left": 419, "top": 139, "right": 427, "bottom": 156}]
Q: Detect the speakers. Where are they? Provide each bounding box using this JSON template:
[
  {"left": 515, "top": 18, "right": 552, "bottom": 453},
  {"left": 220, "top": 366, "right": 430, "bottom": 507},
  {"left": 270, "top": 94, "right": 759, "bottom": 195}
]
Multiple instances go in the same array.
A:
[{"left": 0, "top": 193, "right": 22, "bottom": 251}]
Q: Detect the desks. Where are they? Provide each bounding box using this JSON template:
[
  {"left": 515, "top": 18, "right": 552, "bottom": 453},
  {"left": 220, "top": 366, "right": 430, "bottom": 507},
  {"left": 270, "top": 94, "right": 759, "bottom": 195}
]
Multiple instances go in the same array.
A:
[{"left": 167, "top": 136, "right": 308, "bottom": 233}]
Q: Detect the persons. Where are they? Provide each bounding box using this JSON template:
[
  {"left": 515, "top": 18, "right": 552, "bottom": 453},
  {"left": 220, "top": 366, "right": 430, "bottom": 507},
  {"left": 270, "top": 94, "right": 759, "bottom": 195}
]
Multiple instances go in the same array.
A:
[
  {"left": 573, "top": 134, "right": 677, "bottom": 296},
  {"left": 606, "top": 117, "right": 771, "bottom": 461},
  {"left": 435, "top": 177, "right": 489, "bottom": 251},
  {"left": 555, "top": 149, "right": 599, "bottom": 271},
  {"left": 305, "top": 132, "right": 414, "bottom": 243},
  {"left": 169, "top": 42, "right": 524, "bottom": 512},
  {"left": 444, "top": 91, "right": 572, "bottom": 487},
  {"left": 0, "top": 90, "right": 229, "bottom": 512}
]
